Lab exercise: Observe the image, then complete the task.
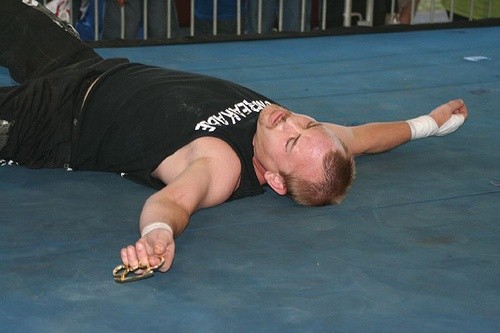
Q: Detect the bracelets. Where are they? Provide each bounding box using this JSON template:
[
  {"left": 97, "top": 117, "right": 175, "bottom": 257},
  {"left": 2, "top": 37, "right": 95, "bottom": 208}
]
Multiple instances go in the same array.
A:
[{"left": 141, "top": 222, "right": 173, "bottom": 239}]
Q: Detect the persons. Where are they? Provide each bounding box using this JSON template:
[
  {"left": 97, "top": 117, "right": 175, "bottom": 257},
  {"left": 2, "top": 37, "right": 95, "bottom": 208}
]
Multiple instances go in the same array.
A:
[{"left": 1, "top": 1, "right": 467, "bottom": 273}]
